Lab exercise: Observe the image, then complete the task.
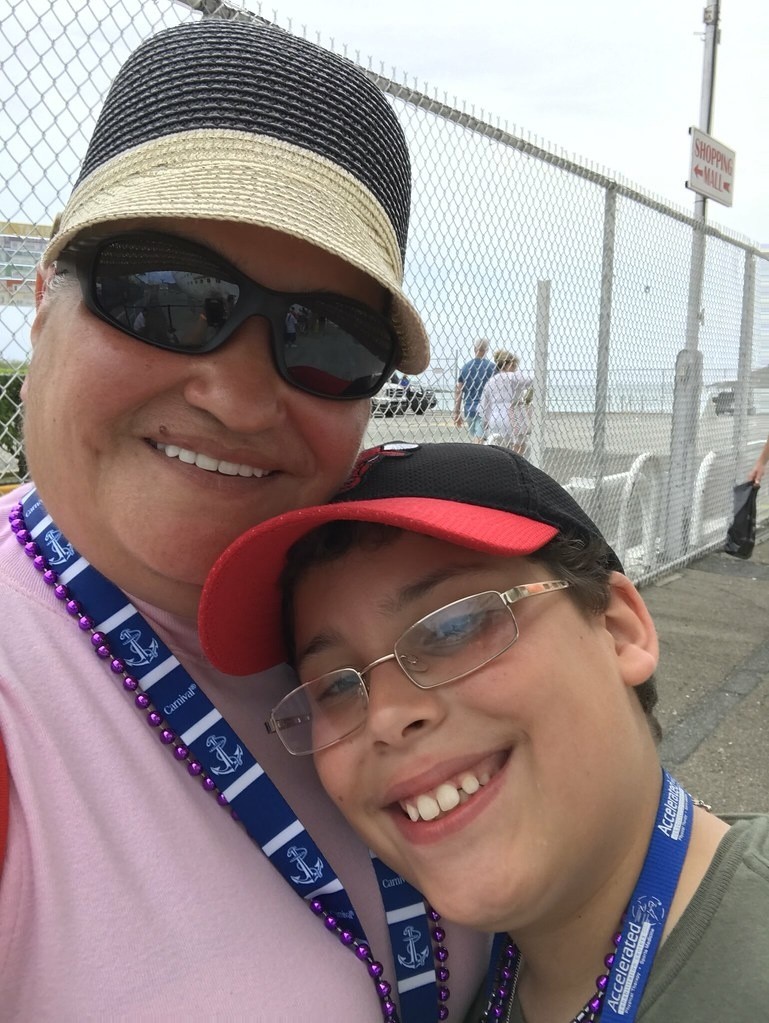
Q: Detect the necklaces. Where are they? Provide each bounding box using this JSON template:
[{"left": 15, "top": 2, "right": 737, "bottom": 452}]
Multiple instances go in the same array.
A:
[
  {"left": 490, "top": 928, "right": 625, "bottom": 1023},
  {"left": 6, "top": 491, "right": 450, "bottom": 1023}
]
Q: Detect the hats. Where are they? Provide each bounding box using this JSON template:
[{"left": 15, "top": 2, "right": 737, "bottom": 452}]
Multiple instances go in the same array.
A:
[
  {"left": 197, "top": 436, "right": 632, "bottom": 676},
  {"left": 34, "top": 18, "right": 433, "bottom": 375}
]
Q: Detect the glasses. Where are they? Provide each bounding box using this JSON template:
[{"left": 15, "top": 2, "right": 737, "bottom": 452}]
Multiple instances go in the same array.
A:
[
  {"left": 261, "top": 579, "right": 571, "bottom": 755},
  {"left": 57, "top": 233, "right": 407, "bottom": 401}
]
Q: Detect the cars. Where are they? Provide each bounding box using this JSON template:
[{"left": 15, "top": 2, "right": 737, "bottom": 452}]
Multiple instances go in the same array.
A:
[
  {"left": 369, "top": 383, "right": 406, "bottom": 417},
  {"left": 712, "top": 392, "right": 755, "bottom": 416},
  {"left": 396, "top": 385, "right": 437, "bottom": 415}
]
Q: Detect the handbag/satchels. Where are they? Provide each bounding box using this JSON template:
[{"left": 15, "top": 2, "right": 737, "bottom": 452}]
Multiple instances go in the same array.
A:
[{"left": 723, "top": 481, "right": 761, "bottom": 560}]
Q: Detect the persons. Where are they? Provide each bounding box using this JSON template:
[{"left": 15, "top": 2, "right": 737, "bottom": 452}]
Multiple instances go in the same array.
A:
[
  {"left": 390, "top": 372, "right": 399, "bottom": 385},
  {"left": 454, "top": 338, "right": 494, "bottom": 445},
  {"left": 132, "top": 294, "right": 236, "bottom": 335},
  {"left": 477, "top": 348, "right": 534, "bottom": 455},
  {"left": 747, "top": 437, "right": 768, "bottom": 483},
  {"left": 288, "top": 438, "right": 768, "bottom": 1023},
  {"left": 0, "top": 22, "right": 494, "bottom": 1023},
  {"left": 286, "top": 304, "right": 327, "bottom": 346},
  {"left": 401, "top": 373, "right": 409, "bottom": 387}
]
